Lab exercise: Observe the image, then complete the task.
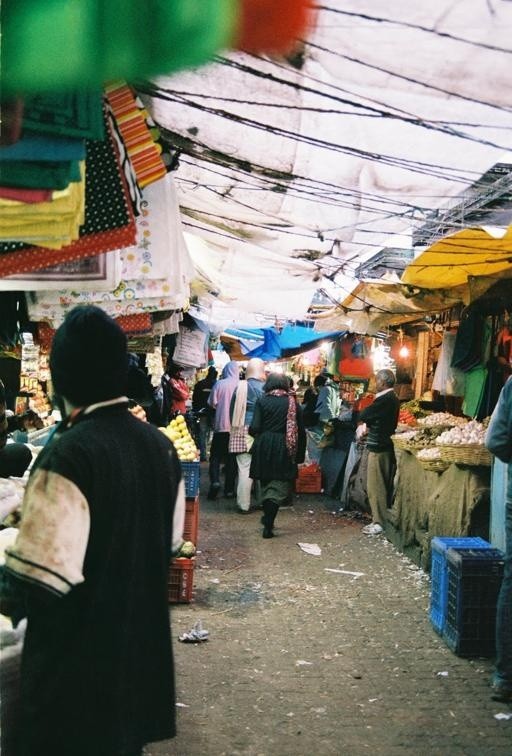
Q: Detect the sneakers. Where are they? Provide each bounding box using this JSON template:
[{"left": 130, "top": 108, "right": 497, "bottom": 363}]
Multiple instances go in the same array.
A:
[
  {"left": 207, "top": 479, "right": 235, "bottom": 498},
  {"left": 490, "top": 676, "right": 512, "bottom": 700},
  {"left": 261, "top": 516, "right": 274, "bottom": 538},
  {"left": 361, "top": 522, "right": 383, "bottom": 535}
]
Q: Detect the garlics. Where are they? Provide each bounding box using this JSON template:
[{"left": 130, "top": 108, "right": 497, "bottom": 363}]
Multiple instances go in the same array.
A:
[{"left": 394, "top": 413, "right": 488, "bottom": 459}]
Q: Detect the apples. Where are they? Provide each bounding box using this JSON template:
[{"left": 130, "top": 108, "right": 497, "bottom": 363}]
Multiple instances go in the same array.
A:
[{"left": 157, "top": 414, "right": 201, "bottom": 462}]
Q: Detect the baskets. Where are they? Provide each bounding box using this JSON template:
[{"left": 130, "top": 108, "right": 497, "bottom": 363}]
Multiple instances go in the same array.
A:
[
  {"left": 390, "top": 423, "right": 491, "bottom": 472},
  {"left": 296, "top": 463, "right": 321, "bottom": 493},
  {"left": 167, "top": 460, "right": 200, "bottom": 603},
  {"left": 430, "top": 536, "right": 505, "bottom": 657}
]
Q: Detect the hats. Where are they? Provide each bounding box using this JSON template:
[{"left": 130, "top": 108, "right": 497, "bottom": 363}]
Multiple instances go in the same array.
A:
[
  {"left": 171, "top": 365, "right": 183, "bottom": 371},
  {"left": 245, "top": 358, "right": 263, "bottom": 380},
  {"left": 49, "top": 306, "right": 125, "bottom": 393}
]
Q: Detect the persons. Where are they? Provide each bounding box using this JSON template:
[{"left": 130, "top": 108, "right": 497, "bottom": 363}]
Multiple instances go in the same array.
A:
[
  {"left": 485, "top": 374, "right": 512, "bottom": 702},
  {"left": 359, "top": 369, "right": 400, "bottom": 534},
  {"left": 192, "top": 358, "right": 342, "bottom": 538},
  {"left": 165, "top": 363, "right": 189, "bottom": 423},
  {"left": 0, "top": 304, "right": 181, "bottom": 756}
]
